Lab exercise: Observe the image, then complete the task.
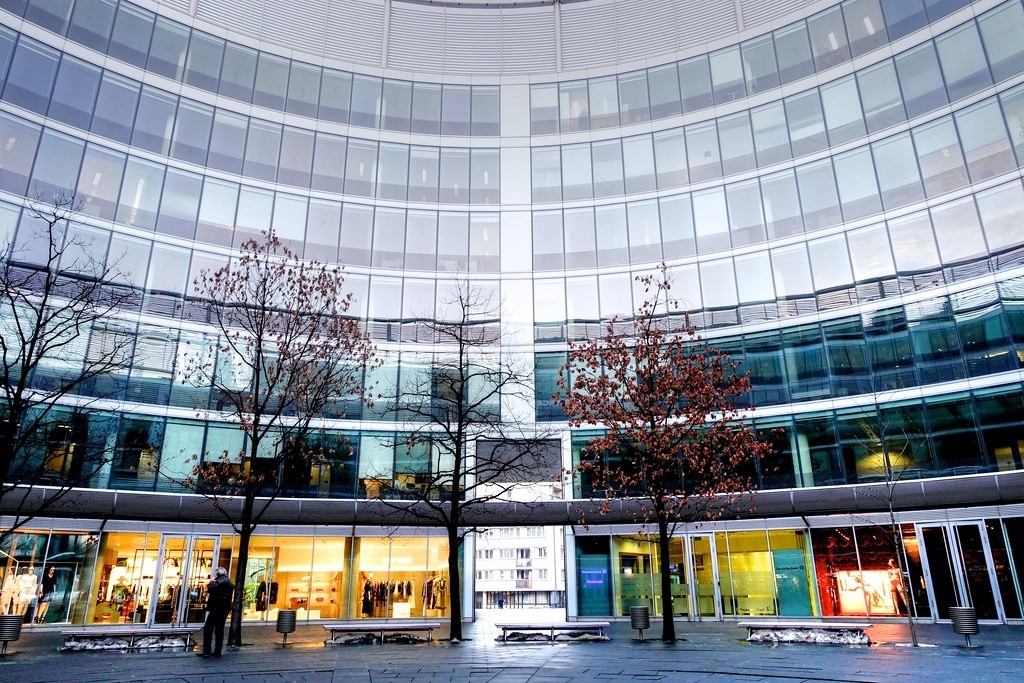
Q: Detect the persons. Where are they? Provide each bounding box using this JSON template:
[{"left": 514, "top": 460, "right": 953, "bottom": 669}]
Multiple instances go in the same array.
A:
[
  {"left": 36, "top": 567, "right": 57, "bottom": 624},
  {"left": 1, "top": 566, "right": 37, "bottom": 616},
  {"left": 438, "top": 578, "right": 447, "bottom": 608},
  {"left": 887, "top": 559, "right": 907, "bottom": 614},
  {"left": 362, "top": 583, "right": 372, "bottom": 617},
  {"left": 498, "top": 597, "right": 504, "bottom": 608},
  {"left": 256, "top": 575, "right": 268, "bottom": 620},
  {"left": 197, "top": 567, "right": 234, "bottom": 658},
  {"left": 268, "top": 575, "right": 279, "bottom": 609}
]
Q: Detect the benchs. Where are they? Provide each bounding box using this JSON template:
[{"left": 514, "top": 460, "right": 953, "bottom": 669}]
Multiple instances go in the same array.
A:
[
  {"left": 737, "top": 621, "right": 875, "bottom": 646},
  {"left": 62, "top": 628, "right": 203, "bottom": 652},
  {"left": 323, "top": 623, "right": 441, "bottom": 646},
  {"left": 495, "top": 622, "right": 610, "bottom": 641}
]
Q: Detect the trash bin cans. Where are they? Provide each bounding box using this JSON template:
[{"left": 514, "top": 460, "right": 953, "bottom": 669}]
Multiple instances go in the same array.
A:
[
  {"left": 0, "top": 615, "right": 23, "bottom": 654},
  {"left": 276, "top": 610, "right": 297, "bottom": 644},
  {"left": 631, "top": 606, "right": 650, "bottom": 639},
  {"left": 948, "top": 607, "right": 980, "bottom": 647}
]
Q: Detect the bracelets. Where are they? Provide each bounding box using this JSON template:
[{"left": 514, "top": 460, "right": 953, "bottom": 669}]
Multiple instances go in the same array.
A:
[{"left": 40, "top": 592, "right": 43, "bottom": 594}]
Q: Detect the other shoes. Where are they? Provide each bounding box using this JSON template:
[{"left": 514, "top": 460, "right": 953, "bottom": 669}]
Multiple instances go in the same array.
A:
[
  {"left": 194, "top": 653, "right": 210, "bottom": 658},
  {"left": 37, "top": 617, "right": 45, "bottom": 621},
  {"left": 211, "top": 652, "right": 222, "bottom": 658}
]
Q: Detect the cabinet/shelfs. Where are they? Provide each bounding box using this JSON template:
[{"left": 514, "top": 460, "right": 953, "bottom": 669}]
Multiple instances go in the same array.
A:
[
  {"left": 125, "top": 549, "right": 233, "bottom": 621},
  {"left": 285, "top": 572, "right": 342, "bottom": 618}
]
